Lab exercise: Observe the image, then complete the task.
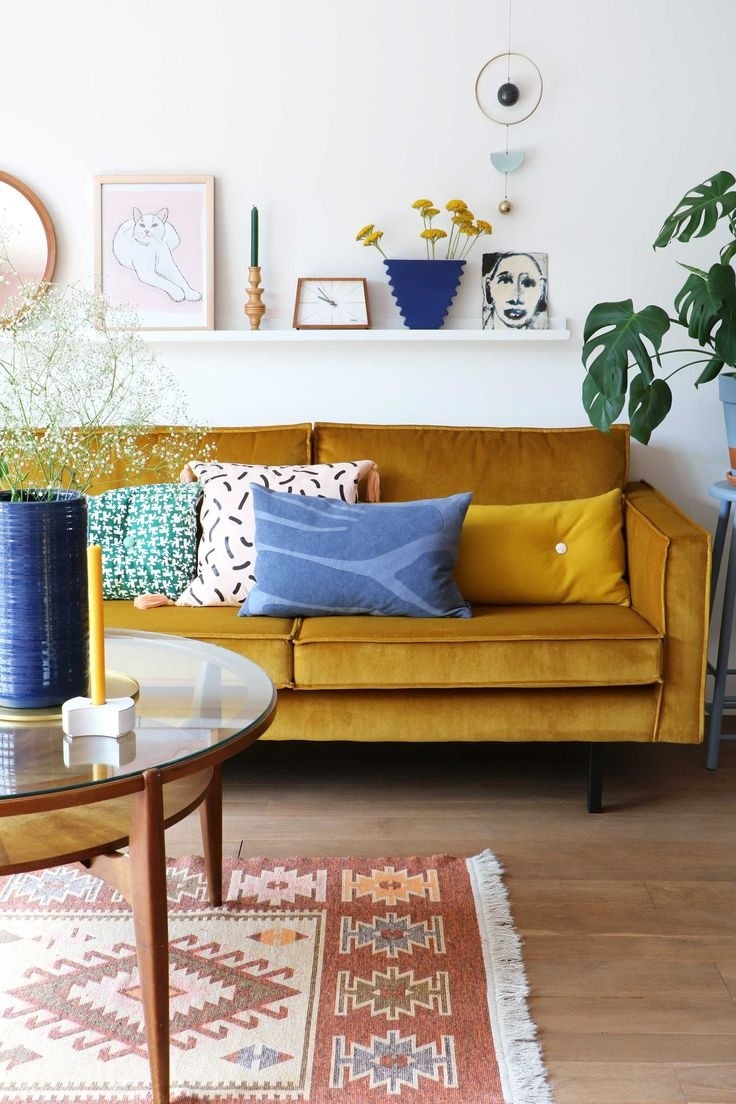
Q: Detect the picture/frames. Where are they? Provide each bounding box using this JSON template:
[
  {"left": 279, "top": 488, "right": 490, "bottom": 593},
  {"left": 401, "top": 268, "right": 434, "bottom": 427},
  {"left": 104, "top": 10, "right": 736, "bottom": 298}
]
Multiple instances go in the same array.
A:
[{"left": 94, "top": 173, "right": 216, "bottom": 331}]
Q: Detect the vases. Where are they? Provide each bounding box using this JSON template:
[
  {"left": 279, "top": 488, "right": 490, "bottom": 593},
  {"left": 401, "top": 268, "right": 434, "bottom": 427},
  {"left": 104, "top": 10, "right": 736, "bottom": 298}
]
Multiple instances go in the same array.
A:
[
  {"left": 0, "top": 488, "right": 88, "bottom": 709},
  {"left": 380, "top": 259, "right": 466, "bottom": 329}
]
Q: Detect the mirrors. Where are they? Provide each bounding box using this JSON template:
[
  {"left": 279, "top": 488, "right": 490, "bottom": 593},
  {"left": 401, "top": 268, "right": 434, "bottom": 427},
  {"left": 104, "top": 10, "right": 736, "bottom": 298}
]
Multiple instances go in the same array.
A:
[{"left": 0, "top": 172, "right": 55, "bottom": 328}]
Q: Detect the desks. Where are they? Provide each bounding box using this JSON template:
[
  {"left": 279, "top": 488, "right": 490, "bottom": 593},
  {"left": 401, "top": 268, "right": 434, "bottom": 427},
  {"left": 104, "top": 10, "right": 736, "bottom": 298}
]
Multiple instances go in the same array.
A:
[{"left": 688, "top": 481, "right": 736, "bottom": 777}]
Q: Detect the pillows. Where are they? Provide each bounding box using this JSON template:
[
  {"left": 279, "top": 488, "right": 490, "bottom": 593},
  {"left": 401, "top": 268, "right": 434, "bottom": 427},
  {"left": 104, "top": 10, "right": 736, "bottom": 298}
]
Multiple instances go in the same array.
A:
[
  {"left": 458, "top": 490, "right": 632, "bottom": 609},
  {"left": 237, "top": 492, "right": 486, "bottom": 618},
  {"left": 87, "top": 484, "right": 211, "bottom": 600},
  {"left": 174, "top": 458, "right": 383, "bottom": 608}
]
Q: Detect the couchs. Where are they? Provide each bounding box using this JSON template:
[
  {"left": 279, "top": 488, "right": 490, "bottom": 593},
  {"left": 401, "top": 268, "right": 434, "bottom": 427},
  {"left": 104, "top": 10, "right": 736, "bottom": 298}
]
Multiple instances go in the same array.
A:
[{"left": 1, "top": 420, "right": 710, "bottom": 816}]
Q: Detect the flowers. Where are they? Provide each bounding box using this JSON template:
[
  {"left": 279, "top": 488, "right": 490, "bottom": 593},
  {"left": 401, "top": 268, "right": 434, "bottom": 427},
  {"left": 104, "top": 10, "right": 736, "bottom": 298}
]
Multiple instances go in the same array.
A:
[
  {"left": 1, "top": 248, "right": 221, "bottom": 502},
  {"left": 356, "top": 201, "right": 493, "bottom": 262}
]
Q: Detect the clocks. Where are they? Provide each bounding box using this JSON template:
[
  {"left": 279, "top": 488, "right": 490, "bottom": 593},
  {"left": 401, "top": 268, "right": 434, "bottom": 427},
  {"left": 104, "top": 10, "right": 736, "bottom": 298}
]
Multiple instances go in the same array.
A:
[{"left": 292, "top": 277, "right": 372, "bottom": 331}]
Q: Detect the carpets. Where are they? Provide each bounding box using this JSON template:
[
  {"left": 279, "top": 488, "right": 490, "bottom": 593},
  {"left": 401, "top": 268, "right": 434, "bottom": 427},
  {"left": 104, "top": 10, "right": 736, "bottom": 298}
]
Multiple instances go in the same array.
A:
[{"left": 0, "top": 845, "right": 553, "bottom": 1104}]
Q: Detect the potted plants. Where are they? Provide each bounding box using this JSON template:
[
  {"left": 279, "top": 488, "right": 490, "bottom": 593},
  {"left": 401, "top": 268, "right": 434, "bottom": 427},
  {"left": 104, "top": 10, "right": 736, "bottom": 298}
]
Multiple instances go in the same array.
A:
[{"left": 580, "top": 169, "right": 736, "bottom": 486}]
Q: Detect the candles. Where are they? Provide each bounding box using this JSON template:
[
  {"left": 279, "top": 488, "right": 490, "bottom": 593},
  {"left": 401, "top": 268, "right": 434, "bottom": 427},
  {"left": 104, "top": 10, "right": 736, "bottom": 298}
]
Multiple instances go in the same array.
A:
[
  {"left": 251, "top": 206, "right": 259, "bottom": 265},
  {"left": 87, "top": 541, "right": 106, "bottom": 704}
]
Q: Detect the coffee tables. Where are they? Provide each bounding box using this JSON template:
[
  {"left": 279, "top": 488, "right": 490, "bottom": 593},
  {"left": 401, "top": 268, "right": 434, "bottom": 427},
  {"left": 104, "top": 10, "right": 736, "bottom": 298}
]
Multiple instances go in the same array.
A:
[{"left": 0, "top": 635, "right": 271, "bottom": 1104}]
